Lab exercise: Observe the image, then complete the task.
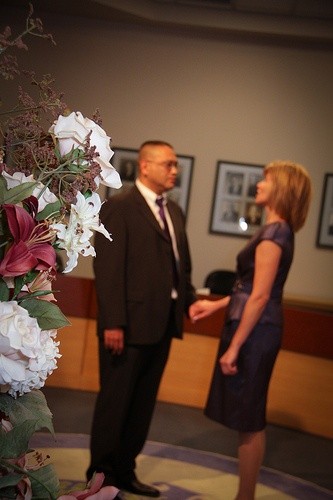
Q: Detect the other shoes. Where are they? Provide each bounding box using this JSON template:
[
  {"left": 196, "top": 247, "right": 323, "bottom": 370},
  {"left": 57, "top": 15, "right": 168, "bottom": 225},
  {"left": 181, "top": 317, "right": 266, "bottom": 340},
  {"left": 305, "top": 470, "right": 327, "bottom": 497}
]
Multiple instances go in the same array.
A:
[
  {"left": 116, "top": 472, "right": 161, "bottom": 498},
  {"left": 85, "top": 466, "right": 124, "bottom": 500}
]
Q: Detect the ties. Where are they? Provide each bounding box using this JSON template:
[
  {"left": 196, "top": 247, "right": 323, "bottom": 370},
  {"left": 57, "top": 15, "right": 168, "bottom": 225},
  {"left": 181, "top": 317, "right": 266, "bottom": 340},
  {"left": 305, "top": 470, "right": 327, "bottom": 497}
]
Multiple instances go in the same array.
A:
[{"left": 156, "top": 196, "right": 181, "bottom": 292}]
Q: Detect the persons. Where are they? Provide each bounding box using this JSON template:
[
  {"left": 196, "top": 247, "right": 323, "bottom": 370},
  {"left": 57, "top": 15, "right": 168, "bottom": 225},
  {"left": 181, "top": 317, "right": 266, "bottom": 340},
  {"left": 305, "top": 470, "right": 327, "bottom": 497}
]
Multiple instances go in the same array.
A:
[
  {"left": 190, "top": 161, "right": 312, "bottom": 500},
  {"left": 222, "top": 174, "right": 261, "bottom": 226},
  {"left": 86, "top": 140, "right": 198, "bottom": 497}
]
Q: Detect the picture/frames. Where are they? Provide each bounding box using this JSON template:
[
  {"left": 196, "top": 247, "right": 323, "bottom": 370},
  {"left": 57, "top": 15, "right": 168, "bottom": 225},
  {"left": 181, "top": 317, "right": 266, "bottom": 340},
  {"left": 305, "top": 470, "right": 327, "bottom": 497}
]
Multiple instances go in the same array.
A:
[
  {"left": 209, "top": 161, "right": 265, "bottom": 239},
  {"left": 106, "top": 146, "right": 195, "bottom": 217},
  {"left": 316, "top": 173, "right": 333, "bottom": 250}
]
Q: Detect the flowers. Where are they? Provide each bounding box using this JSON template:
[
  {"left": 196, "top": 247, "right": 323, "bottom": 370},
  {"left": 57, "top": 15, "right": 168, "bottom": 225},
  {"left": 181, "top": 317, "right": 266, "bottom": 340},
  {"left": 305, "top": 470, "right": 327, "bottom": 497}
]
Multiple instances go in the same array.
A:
[{"left": 0, "top": 0, "right": 122, "bottom": 500}]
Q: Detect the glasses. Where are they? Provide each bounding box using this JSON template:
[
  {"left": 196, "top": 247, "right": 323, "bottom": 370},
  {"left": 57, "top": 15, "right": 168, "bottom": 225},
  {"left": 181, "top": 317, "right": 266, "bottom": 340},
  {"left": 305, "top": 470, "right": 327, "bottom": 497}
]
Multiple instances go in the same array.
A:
[{"left": 156, "top": 160, "right": 178, "bottom": 170}]
